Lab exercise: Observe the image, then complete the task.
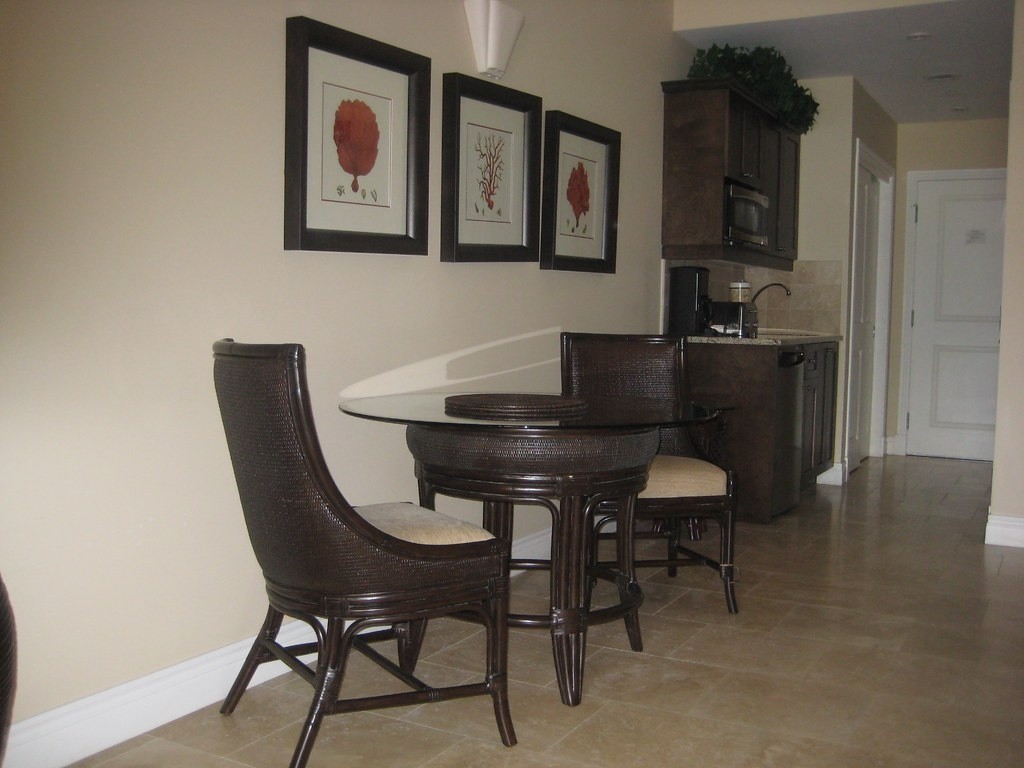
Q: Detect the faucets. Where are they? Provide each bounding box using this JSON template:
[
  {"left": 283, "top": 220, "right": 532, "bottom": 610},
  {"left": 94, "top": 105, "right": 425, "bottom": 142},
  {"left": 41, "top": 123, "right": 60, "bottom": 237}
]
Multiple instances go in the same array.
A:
[{"left": 751, "top": 281, "right": 792, "bottom": 304}]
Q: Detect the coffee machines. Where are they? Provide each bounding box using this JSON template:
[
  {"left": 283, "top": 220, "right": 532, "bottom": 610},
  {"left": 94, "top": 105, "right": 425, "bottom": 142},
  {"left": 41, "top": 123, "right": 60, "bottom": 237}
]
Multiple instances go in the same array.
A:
[{"left": 666, "top": 266, "right": 715, "bottom": 338}]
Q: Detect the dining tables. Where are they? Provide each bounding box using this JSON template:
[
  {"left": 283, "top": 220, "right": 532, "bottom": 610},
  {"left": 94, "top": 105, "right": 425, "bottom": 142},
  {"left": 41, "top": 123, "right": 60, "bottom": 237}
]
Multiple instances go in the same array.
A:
[{"left": 338, "top": 384, "right": 720, "bottom": 707}]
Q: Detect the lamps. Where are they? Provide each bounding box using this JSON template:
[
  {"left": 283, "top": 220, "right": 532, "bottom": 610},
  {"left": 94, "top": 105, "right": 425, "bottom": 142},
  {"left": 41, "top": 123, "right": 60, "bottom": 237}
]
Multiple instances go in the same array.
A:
[{"left": 462, "top": 0, "right": 525, "bottom": 79}]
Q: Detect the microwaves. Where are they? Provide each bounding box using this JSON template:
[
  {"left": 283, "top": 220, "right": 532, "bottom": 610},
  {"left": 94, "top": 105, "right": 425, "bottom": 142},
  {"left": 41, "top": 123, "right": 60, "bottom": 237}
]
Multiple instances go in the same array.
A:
[{"left": 725, "top": 183, "right": 771, "bottom": 247}]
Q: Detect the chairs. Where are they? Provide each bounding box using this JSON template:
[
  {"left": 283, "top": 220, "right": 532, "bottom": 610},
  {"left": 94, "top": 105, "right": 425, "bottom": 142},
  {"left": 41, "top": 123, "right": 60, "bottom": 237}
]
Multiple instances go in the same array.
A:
[
  {"left": 209, "top": 335, "right": 520, "bottom": 767},
  {"left": 558, "top": 328, "right": 740, "bottom": 618}
]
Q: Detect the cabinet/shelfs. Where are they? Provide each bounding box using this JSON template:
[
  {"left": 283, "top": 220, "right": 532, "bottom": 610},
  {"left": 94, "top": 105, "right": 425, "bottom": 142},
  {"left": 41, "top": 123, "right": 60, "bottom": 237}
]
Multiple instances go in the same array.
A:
[
  {"left": 802, "top": 343, "right": 840, "bottom": 484},
  {"left": 661, "top": 76, "right": 801, "bottom": 272}
]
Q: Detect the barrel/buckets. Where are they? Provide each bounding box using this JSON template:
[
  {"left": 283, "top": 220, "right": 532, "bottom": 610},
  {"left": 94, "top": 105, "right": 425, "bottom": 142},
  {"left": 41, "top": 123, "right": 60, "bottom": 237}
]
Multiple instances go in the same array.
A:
[{"left": 729, "top": 280, "right": 752, "bottom": 303}]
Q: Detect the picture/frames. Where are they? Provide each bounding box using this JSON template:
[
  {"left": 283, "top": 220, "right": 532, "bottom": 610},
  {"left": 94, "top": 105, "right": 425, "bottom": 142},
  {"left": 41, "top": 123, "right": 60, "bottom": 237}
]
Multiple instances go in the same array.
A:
[
  {"left": 441, "top": 73, "right": 543, "bottom": 265},
  {"left": 539, "top": 110, "right": 622, "bottom": 275},
  {"left": 284, "top": 15, "right": 432, "bottom": 258}
]
